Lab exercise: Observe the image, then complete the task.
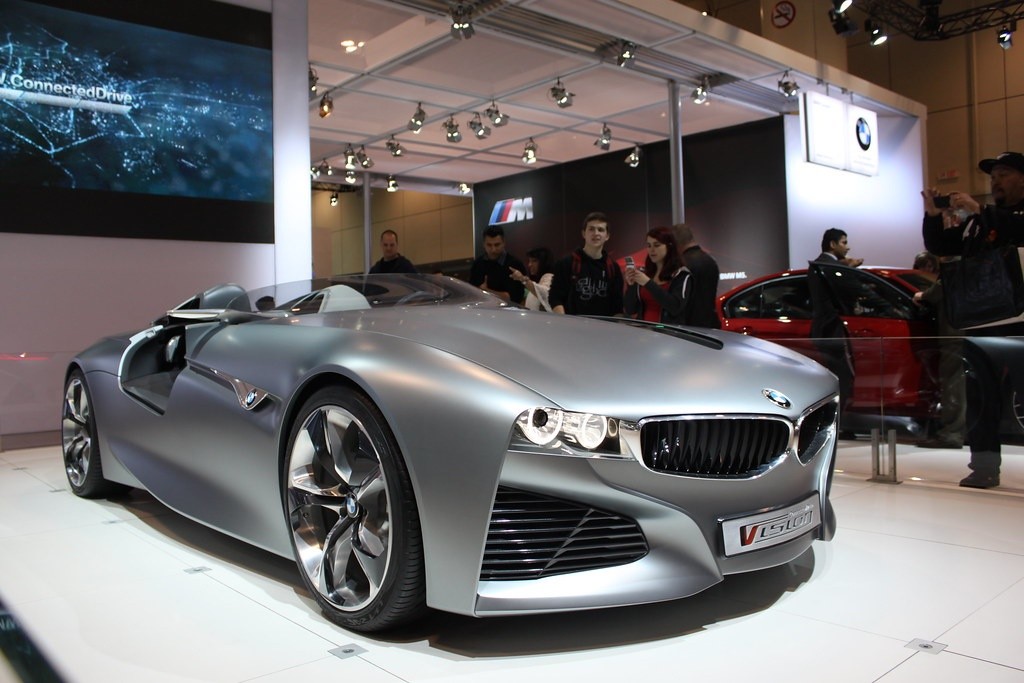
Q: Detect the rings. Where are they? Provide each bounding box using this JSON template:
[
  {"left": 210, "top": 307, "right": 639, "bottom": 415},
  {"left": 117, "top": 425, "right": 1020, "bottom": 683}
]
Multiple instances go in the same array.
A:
[{"left": 956, "top": 196, "right": 960, "bottom": 199}]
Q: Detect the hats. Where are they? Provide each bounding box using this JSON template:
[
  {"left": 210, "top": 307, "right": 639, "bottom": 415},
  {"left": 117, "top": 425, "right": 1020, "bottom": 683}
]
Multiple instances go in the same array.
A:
[{"left": 978, "top": 152, "right": 1023, "bottom": 174}]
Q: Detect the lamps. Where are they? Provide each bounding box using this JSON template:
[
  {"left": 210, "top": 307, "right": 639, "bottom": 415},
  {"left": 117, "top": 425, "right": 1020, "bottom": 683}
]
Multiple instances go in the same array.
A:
[
  {"left": 997, "top": 26, "right": 1012, "bottom": 49},
  {"left": 864, "top": 16, "right": 888, "bottom": 45},
  {"left": 311, "top": 0, "right": 860, "bottom": 207}
]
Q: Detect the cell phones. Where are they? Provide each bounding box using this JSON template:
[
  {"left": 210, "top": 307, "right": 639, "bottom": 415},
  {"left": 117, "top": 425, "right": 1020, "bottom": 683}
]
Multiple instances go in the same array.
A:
[
  {"left": 933, "top": 195, "right": 949, "bottom": 208},
  {"left": 625, "top": 257, "right": 635, "bottom": 271}
]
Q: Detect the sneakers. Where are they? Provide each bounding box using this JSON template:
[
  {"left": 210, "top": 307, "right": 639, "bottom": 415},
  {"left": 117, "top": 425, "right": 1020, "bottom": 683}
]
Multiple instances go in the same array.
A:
[{"left": 959, "top": 471, "right": 1000, "bottom": 488}]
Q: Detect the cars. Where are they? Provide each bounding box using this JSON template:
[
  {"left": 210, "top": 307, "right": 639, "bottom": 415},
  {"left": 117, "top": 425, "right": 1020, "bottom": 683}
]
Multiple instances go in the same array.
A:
[{"left": 646, "top": 265, "right": 1024, "bottom": 441}]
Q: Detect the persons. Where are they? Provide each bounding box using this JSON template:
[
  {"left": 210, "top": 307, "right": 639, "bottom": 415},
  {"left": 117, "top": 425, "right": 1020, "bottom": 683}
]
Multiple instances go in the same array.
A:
[
  {"left": 913, "top": 149, "right": 1024, "bottom": 489},
  {"left": 807, "top": 228, "right": 864, "bottom": 439},
  {"left": 623, "top": 227, "right": 694, "bottom": 325},
  {"left": 548, "top": 211, "right": 628, "bottom": 324},
  {"left": 368, "top": 231, "right": 417, "bottom": 274},
  {"left": 509, "top": 246, "right": 553, "bottom": 310},
  {"left": 470, "top": 225, "right": 524, "bottom": 304},
  {"left": 670, "top": 223, "right": 722, "bottom": 329}
]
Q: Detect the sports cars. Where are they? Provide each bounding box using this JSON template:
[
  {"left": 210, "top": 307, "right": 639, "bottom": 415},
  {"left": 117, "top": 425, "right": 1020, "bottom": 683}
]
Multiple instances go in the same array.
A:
[{"left": 60, "top": 272, "right": 839, "bottom": 636}]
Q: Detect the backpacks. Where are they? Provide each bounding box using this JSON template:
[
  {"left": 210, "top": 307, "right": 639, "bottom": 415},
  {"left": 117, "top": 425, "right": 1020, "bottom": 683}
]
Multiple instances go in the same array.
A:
[{"left": 940, "top": 249, "right": 1015, "bottom": 328}]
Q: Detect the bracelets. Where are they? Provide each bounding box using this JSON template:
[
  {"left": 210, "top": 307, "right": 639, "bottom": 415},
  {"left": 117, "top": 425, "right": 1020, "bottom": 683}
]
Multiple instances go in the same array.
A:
[{"left": 521, "top": 275, "right": 530, "bottom": 285}]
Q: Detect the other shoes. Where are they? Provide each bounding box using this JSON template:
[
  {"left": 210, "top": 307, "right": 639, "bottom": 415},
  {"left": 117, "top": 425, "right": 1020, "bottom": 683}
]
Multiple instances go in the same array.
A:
[
  {"left": 839, "top": 430, "right": 857, "bottom": 441},
  {"left": 917, "top": 439, "right": 946, "bottom": 448}
]
